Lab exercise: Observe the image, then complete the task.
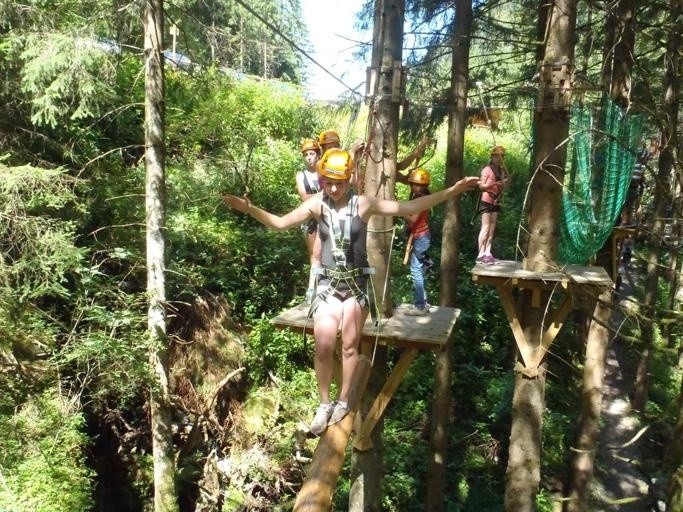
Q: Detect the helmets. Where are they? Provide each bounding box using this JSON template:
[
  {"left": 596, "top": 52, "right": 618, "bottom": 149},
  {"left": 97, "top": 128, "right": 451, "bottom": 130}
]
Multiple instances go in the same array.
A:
[
  {"left": 301, "top": 140, "right": 320, "bottom": 152},
  {"left": 319, "top": 148, "right": 352, "bottom": 180},
  {"left": 491, "top": 146, "right": 505, "bottom": 155},
  {"left": 319, "top": 130, "right": 340, "bottom": 145},
  {"left": 408, "top": 170, "right": 430, "bottom": 184}
]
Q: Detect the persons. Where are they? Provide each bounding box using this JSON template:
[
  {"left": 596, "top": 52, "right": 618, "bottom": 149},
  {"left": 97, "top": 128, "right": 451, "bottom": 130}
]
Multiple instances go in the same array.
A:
[
  {"left": 400, "top": 168, "right": 433, "bottom": 316},
  {"left": 295, "top": 141, "right": 326, "bottom": 305},
  {"left": 474, "top": 144, "right": 511, "bottom": 265},
  {"left": 320, "top": 132, "right": 365, "bottom": 186},
  {"left": 220, "top": 148, "right": 481, "bottom": 435}
]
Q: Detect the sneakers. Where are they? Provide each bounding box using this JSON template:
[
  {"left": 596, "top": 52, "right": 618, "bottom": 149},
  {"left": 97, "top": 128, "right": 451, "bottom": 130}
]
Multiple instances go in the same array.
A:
[
  {"left": 404, "top": 304, "right": 429, "bottom": 317},
  {"left": 310, "top": 405, "right": 333, "bottom": 433},
  {"left": 327, "top": 403, "right": 350, "bottom": 426},
  {"left": 475, "top": 255, "right": 500, "bottom": 265},
  {"left": 306, "top": 289, "right": 313, "bottom": 305}
]
